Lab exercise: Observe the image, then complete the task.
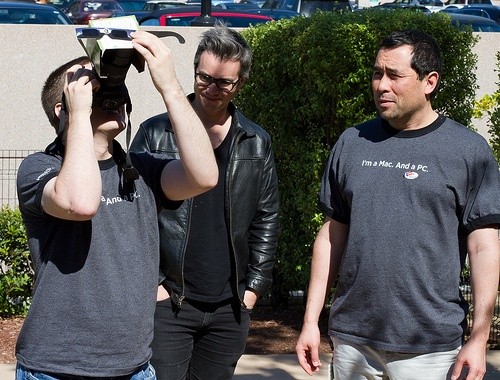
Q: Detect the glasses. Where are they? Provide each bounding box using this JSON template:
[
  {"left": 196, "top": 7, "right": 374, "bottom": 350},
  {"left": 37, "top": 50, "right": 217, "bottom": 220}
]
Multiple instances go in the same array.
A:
[{"left": 194, "top": 65, "right": 241, "bottom": 93}]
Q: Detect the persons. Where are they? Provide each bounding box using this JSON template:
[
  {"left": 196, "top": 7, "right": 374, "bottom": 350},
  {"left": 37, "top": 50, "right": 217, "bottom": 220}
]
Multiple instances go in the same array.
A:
[
  {"left": 295, "top": 30, "right": 500, "bottom": 380},
  {"left": 15, "top": 29, "right": 220, "bottom": 380},
  {"left": 127, "top": 19, "right": 281, "bottom": 380}
]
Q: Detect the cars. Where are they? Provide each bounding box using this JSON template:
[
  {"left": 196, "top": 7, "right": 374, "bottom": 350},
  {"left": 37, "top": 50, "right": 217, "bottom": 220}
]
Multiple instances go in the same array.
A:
[{"left": 0, "top": 1, "right": 500, "bottom": 33}]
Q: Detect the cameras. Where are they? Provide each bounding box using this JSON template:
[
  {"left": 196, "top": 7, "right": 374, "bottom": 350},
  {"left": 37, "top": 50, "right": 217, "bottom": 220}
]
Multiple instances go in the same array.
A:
[{"left": 92, "top": 46, "right": 145, "bottom": 113}]
